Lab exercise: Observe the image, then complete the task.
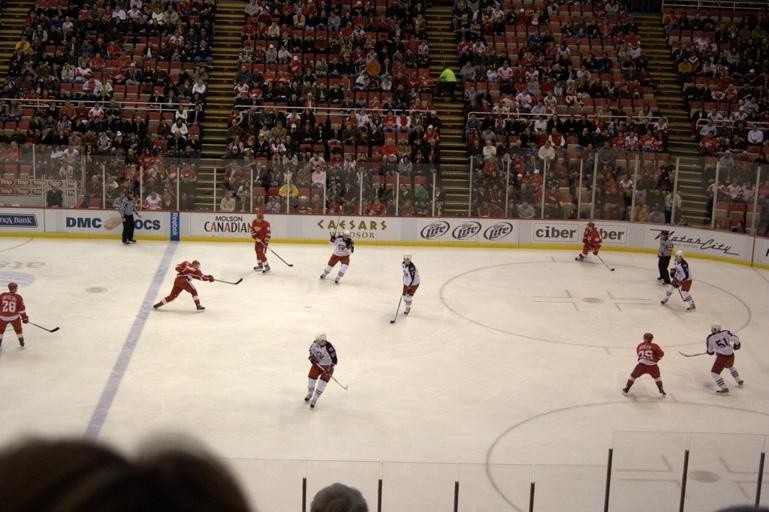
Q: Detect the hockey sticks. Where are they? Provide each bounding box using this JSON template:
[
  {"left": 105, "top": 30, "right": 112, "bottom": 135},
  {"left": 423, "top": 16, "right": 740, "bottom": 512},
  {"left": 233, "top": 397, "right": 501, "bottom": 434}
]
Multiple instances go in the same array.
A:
[
  {"left": 201, "top": 275, "right": 244, "bottom": 285},
  {"left": 28, "top": 321, "right": 61, "bottom": 332},
  {"left": 390, "top": 291, "right": 403, "bottom": 323},
  {"left": 315, "top": 363, "right": 349, "bottom": 390},
  {"left": 334, "top": 205, "right": 343, "bottom": 238},
  {"left": 596, "top": 253, "right": 616, "bottom": 271},
  {"left": 672, "top": 274, "right": 690, "bottom": 302},
  {"left": 678, "top": 351, "right": 707, "bottom": 358},
  {"left": 260, "top": 241, "right": 294, "bottom": 267}
]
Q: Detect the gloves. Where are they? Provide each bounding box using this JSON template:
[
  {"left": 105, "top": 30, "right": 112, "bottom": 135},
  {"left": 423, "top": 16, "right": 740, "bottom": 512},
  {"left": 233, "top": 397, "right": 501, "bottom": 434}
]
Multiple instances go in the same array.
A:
[
  {"left": 23, "top": 316, "right": 29, "bottom": 324},
  {"left": 732, "top": 343, "right": 741, "bottom": 350},
  {"left": 669, "top": 269, "right": 677, "bottom": 278},
  {"left": 581, "top": 238, "right": 588, "bottom": 245},
  {"left": 324, "top": 369, "right": 334, "bottom": 376},
  {"left": 331, "top": 235, "right": 336, "bottom": 242},
  {"left": 207, "top": 275, "right": 216, "bottom": 282},
  {"left": 593, "top": 248, "right": 599, "bottom": 255},
  {"left": 256, "top": 236, "right": 268, "bottom": 249},
  {"left": 677, "top": 281, "right": 683, "bottom": 288},
  {"left": 309, "top": 355, "right": 320, "bottom": 366}
]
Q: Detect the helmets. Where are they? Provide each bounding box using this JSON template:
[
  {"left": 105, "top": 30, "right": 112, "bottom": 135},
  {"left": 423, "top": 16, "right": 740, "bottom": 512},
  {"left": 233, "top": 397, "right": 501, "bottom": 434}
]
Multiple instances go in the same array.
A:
[
  {"left": 343, "top": 231, "right": 350, "bottom": 236},
  {"left": 711, "top": 322, "right": 722, "bottom": 333},
  {"left": 643, "top": 332, "right": 653, "bottom": 343},
  {"left": 587, "top": 222, "right": 596, "bottom": 227},
  {"left": 257, "top": 213, "right": 264, "bottom": 218},
  {"left": 403, "top": 251, "right": 413, "bottom": 260},
  {"left": 7, "top": 282, "right": 19, "bottom": 289},
  {"left": 314, "top": 331, "right": 328, "bottom": 340},
  {"left": 674, "top": 249, "right": 684, "bottom": 258},
  {"left": 192, "top": 260, "right": 200, "bottom": 266}
]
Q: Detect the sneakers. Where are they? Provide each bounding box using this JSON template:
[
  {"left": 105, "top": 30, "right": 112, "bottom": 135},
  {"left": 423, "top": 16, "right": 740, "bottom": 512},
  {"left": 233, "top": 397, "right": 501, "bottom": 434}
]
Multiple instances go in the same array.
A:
[
  {"left": 656, "top": 277, "right": 672, "bottom": 286},
  {"left": 404, "top": 308, "right": 411, "bottom": 314},
  {"left": 305, "top": 392, "right": 318, "bottom": 408},
  {"left": 716, "top": 388, "right": 730, "bottom": 394},
  {"left": 153, "top": 304, "right": 158, "bottom": 309},
  {"left": 737, "top": 380, "right": 744, "bottom": 385},
  {"left": 253, "top": 263, "right": 272, "bottom": 274},
  {"left": 686, "top": 304, "right": 696, "bottom": 310},
  {"left": 196, "top": 306, "right": 207, "bottom": 310},
  {"left": 661, "top": 297, "right": 669, "bottom": 304},
  {"left": 121, "top": 239, "right": 137, "bottom": 244},
  {"left": 575, "top": 254, "right": 585, "bottom": 260},
  {"left": 19, "top": 340, "right": 25, "bottom": 347},
  {"left": 624, "top": 387, "right": 629, "bottom": 393},
  {"left": 319, "top": 272, "right": 341, "bottom": 284},
  {"left": 660, "top": 390, "right": 667, "bottom": 395}
]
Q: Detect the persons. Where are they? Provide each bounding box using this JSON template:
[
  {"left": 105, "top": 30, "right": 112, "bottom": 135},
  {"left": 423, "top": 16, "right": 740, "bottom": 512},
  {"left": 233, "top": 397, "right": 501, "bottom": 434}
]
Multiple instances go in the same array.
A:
[
  {"left": 303, "top": 331, "right": 338, "bottom": 408},
  {"left": 705, "top": 321, "right": 744, "bottom": 395},
  {"left": 659, "top": 250, "right": 696, "bottom": 310},
  {"left": 574, "top": 222, "right": 602, "bottom": 262},
  {"left": 0, "top": 282, "right": 29, "bottom": 350},
  {"left": 152, "top": 259, "right": 214, "bottom": 312},
  {"left": 2, "top": 1, "right": 768, "bottom": 244},
  {"left": 655, "top": 231, "right": 674, "bottom": 286},
  {"left": 621, "top": 332, "right": 666, "bottom": 396},
  {"left": 249, "top": 212, "right": 273, "bottom": 274},
  {"left": 319, "top": 230, "right": 354, "bottom": 285},
  {"left": 401, "top": 252, "right": 421, "bottom": 316}
]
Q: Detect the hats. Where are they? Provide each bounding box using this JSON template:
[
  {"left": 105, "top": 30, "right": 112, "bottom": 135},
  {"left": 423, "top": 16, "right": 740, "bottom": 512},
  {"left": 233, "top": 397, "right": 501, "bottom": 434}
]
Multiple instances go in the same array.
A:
[{"left": 661, "top": 230, "right": 670, "bottom": 235}]
